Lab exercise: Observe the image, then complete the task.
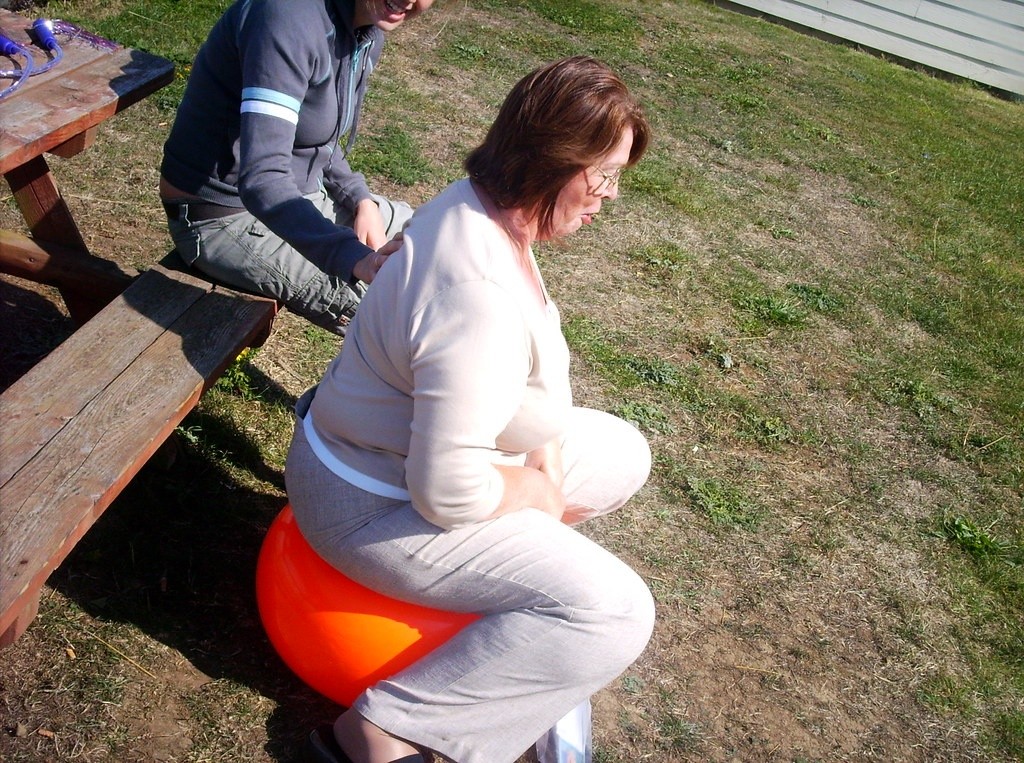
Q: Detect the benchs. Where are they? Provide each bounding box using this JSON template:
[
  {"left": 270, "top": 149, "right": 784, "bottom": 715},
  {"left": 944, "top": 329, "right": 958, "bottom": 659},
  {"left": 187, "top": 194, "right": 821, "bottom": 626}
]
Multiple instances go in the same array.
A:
[{"left": 0, "top": 247, "right": 284, "bottom": 652}]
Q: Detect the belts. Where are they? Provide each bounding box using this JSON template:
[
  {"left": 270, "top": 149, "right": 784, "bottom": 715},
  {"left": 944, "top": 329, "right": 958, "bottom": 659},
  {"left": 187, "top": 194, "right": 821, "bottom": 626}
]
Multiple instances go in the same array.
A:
[{"left": 161, "top": 199, "right": 246, "bottom": 222}]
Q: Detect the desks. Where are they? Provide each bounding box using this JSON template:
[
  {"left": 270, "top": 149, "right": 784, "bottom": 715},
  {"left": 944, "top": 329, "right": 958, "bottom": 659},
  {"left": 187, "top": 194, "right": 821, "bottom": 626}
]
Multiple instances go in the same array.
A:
[{"left": 0, "top": 7, "right": 179, "bottom": 494}]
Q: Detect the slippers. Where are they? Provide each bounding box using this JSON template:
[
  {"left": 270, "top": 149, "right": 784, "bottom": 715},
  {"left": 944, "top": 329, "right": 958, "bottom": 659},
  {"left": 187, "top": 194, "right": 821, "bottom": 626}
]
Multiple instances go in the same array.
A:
[{"left": 310, "top": 724, "right": 435, "bottom": 763}]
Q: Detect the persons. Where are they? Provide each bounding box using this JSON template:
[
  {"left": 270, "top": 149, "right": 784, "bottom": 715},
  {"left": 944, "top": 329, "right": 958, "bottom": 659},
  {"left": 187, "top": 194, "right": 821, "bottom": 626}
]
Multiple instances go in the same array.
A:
[
  {"left": 278, "top": 56, "right": 659, "bottom": 763},
  {"left": 157, "top": 0, "right": 434, "bottom": 422}
]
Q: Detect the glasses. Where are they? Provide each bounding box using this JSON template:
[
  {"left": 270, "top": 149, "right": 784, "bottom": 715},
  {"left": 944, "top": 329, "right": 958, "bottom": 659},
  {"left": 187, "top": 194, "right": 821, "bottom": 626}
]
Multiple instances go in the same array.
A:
[{"left": 593, "top": 164, "right": 623, "bottom": 192}]
